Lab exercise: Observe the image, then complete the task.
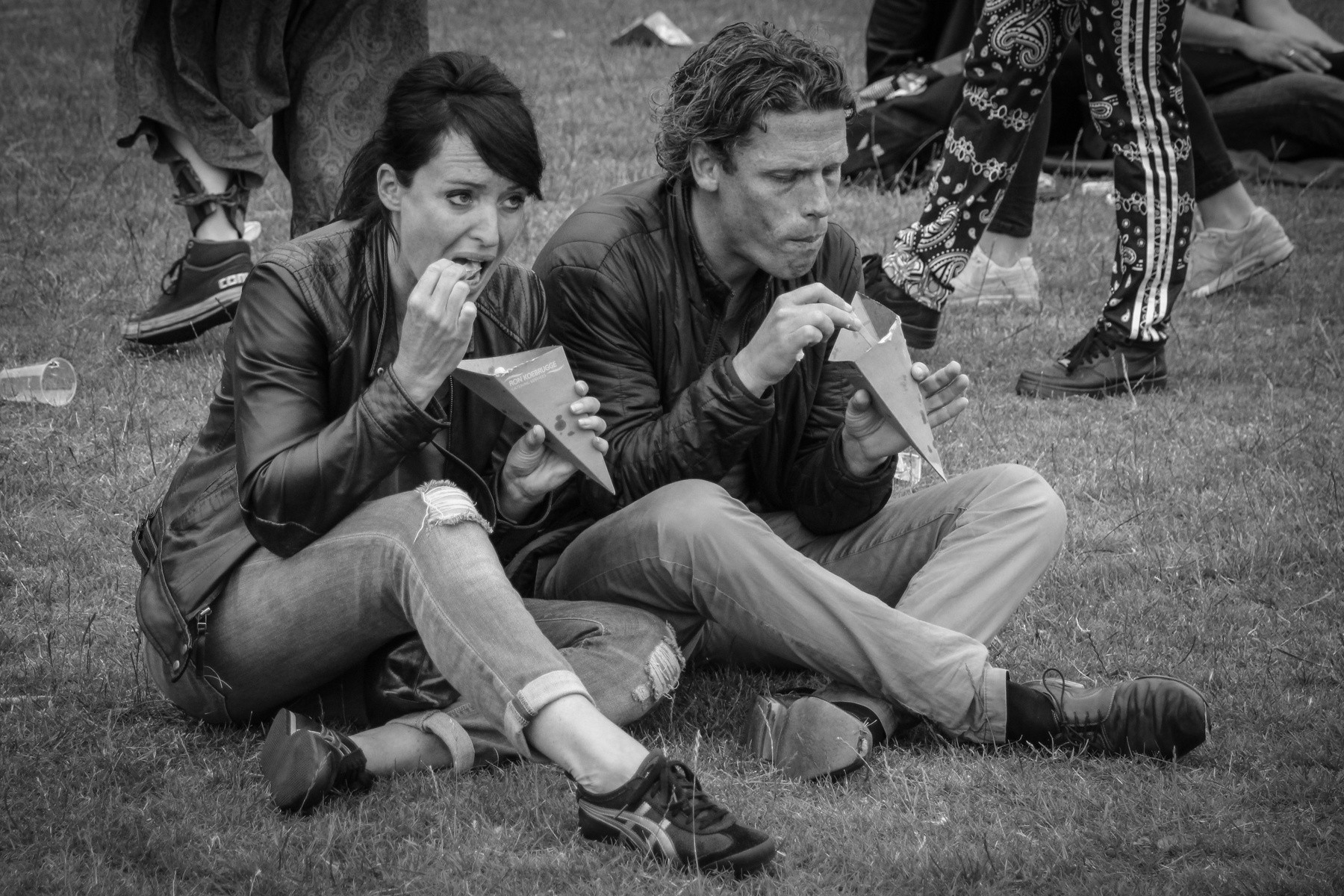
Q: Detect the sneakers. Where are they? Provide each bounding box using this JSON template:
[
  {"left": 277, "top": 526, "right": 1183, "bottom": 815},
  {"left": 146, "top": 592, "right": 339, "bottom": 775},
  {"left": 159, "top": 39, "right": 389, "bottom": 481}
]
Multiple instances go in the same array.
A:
[
  {"left": 1016, "top": 320, "right": 1170, "bottom": 400},
  {"left": 260, "top": 708, "right": 366, "bottom": 812},
  {"left": 575, "top": 750, "right": 776, "bottom": 876},
  {"left": 749, "top": 694, "right": 872, "bottom": 782},
  {"left": 122, "top": 239, "right": 254, "bottom": 344},
  {"left": 945, "top": 245, "right": 1040, "bottom": 306},
  {"left": 1021, "top": 668, "right": 1212, "bottom": 761},
  {"left": 1179, "top": 207, "right": 1294, "bottom": 301},
  {"left": 859, "top": 254, "right": 941, "bottom": 350}
]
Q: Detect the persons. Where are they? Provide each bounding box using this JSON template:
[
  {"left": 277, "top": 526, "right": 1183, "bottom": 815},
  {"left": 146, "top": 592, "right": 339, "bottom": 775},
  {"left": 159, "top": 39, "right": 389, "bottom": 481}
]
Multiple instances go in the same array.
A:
[
  {"left": 134, "top": 46, "right": 777, "bottom": 875},
  {"left": 842, "top": 0, "right": 1344, "bottom": 401},
  {"left": 115, "top": 0, "right": 433, "bottom": 350},
  {"left": 523, "top": 24, "right": 1220, "bottom": 781}
]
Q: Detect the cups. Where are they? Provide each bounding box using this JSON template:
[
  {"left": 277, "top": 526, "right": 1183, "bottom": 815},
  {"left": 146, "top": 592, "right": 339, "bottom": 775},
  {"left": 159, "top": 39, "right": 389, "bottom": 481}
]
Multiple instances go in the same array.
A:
[{"left": 0, "top": 357, "right": 77, "bottom": 407}]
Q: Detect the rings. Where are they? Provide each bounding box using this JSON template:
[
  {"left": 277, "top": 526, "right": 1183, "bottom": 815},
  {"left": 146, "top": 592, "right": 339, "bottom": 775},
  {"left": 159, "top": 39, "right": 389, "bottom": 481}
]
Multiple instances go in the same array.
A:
[{"left": 1286, "top": 49, "right": 1295, "bottom": 58}]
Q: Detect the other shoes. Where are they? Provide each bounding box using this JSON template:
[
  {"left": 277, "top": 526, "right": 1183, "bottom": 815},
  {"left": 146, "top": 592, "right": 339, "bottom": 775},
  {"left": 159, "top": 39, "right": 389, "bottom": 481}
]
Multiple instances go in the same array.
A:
[
  {"left": 1082, "top": 179, "right": 1116, "bottom": 201},
  {"left": 1035, "top": 170, "right": 1062, "bottom": 200}
]
